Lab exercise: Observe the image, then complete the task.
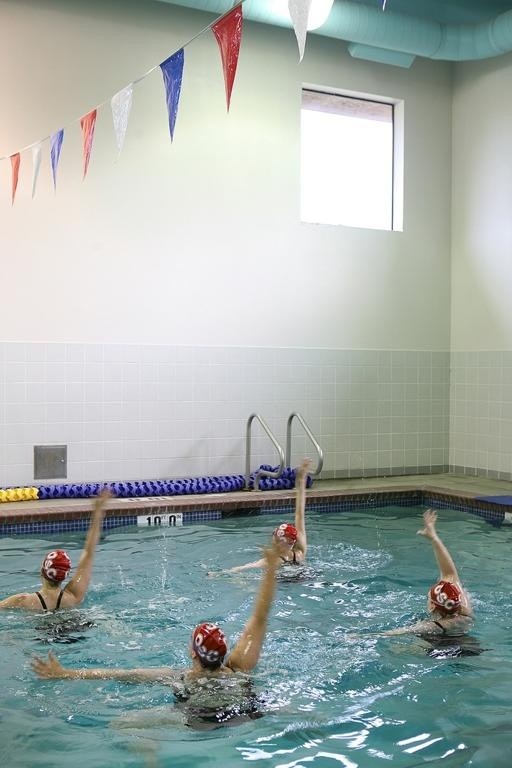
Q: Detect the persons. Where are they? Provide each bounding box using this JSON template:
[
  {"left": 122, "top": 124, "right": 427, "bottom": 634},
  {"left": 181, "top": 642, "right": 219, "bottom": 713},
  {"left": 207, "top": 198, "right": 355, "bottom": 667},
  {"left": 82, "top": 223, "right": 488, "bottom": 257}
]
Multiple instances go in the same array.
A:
[
  {"left": 341, "top": 507, "right": 506, "bottom": 665},
  {"left": 0, "top": 490, "right": 155, "bottom": 646},
  {"left": 27, "top": 537, "right": 288, "bottom": 766},
  {"left": 206, "top": 453, "right": 319, "bottom": 586}
]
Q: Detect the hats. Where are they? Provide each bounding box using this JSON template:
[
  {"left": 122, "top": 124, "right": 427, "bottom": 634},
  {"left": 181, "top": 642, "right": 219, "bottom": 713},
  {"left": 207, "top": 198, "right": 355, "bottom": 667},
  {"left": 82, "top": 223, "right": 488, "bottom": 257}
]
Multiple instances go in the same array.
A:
[
  {"left": 42, "top": 550, "right": 72, "bottom": 581},
  {"left": 273, "top": 523, "right": 298, "bottom": 545},
  {"left": 430, "top": 580, "right": 461, "bottom": 615},
  {"left": 191, "top": 623, "right": 226, "bottom": 664}
]
